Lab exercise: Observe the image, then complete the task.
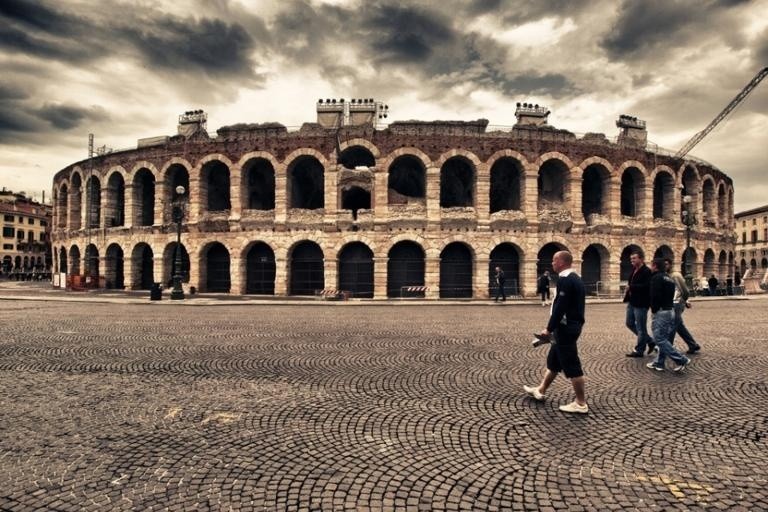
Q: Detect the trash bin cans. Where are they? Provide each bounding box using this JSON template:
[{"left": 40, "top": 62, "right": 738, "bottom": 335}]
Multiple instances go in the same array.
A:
[{"left": 150, "top": 283, "right": 163, "bottom": 300}]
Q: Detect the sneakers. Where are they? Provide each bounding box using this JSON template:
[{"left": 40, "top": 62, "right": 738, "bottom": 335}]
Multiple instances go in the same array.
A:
[
  {"left": 523, "top": 385, "right": 546, "bottom": 401},
  {"left": 626, "top": 343, "right": 700, "bottom": 372},
  {"left": 559, "top": 401, "right": 588, "bottom": 413}
]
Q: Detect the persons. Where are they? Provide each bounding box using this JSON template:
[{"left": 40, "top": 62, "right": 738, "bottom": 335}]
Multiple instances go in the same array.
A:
[
  {"left": 523, "top": 250, "right": 588, "bottom": 414},
  {"left": 495, "top": 266, "right": 506, "bottom": 301},
  {"left": 708, "top": 275, "right": 717, "bottom": 295},
  {"left": 664, "top": 258, "right": 701, "bottom": 354},
  {"left": 623, "top": 250, "right": 656, "bottom": 357},
  {"left": 725, "top": 275, "right": 732, "bottom": 295},
  {"left": 537, "top": 268, "right": 551, "bottom": 307},
  {"left": 735, "top": 272, "right": 740, "bottom": 286},
  {"left": 646, "top": 257, "right": 691, "bottom": 373}
]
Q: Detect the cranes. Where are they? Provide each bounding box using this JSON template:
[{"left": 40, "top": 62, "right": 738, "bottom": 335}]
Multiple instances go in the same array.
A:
[{"left": 673, "top": 66, "right": 768, "bottom": 164}]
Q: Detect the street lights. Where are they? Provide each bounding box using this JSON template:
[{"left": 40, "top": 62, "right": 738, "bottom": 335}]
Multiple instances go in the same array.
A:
[{"left": 170, "top": 185, "right": 187, "bottom": 301}]
